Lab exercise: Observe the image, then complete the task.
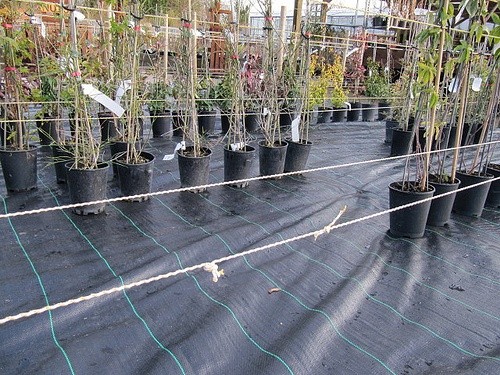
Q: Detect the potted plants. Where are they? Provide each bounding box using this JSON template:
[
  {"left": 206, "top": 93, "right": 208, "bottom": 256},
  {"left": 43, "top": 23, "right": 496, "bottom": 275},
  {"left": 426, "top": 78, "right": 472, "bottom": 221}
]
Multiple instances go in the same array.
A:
[
  {"left": 0, "top": 0, "right": 315, "bottom": 219},
  {"left": 313, "top": 0, "right": 500, "bottom": 240}
]
